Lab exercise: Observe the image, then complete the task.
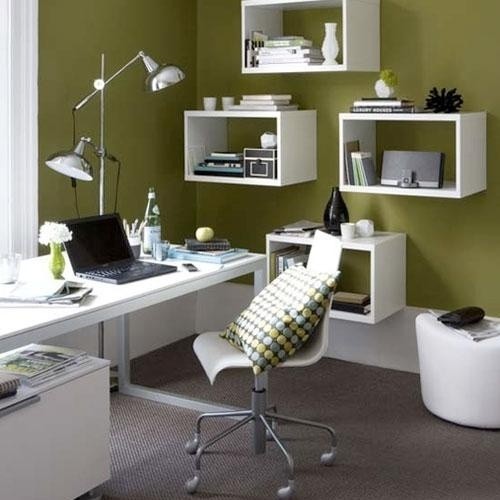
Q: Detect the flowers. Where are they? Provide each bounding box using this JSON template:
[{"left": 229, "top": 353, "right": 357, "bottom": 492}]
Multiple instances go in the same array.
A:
[{"left": 37, "top": 217, "right": 71, "bottom": 247}]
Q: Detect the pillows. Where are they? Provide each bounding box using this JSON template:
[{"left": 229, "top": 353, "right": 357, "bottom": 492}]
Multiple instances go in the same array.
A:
[{"left": 218, "top": 260, "right": 342, "bottom": 377}]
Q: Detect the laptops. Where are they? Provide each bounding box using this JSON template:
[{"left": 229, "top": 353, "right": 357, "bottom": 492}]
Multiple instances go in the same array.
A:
[{"left": 58, "top": 213, "right": 177, "bottom": 285}]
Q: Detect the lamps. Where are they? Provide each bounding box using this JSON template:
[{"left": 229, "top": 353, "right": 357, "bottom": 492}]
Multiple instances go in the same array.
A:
[
  {"left": 71, "top": 50, "right": 187, "bottom": 109},
  {"left": 42, "top": 136, "right": 120, "bottom": 186}
]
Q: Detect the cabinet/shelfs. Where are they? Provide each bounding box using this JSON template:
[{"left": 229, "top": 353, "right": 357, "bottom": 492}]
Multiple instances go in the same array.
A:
[
  {"left": 338, "top": 112, "right": 486, "bottom": 200},
  {"left": 1, "top": 343, "right": 113, "bottom": 500},
  {"left": 239, "top": 0, "right": 381, "bottom": 74},
  {"left": 183, "top": 110, "right": 320, "bottom": 188},
  {"left": 265, "top": 222, "right": 408, "bottom": 327}
]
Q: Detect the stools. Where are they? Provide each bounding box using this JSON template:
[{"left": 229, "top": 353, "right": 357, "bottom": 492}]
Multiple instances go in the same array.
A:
[{"left": 412, "top": 311, "right": 500, "bottom": 430}]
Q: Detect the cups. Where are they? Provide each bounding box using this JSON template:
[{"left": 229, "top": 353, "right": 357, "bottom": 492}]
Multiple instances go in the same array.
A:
[
  {"left": 0, "top": 257, "right": 18, "bottom": 285},
  {"left": 153, "top": 241, "right": 169, "bottom": 261},
  {"left": 340, "top": 222, "right": 357, "bottom": 241},
  {"left": 355, "top": 219, "right": 375, "bottom": 236},
  {"left": 202, "top": 97, "right": 216, "bottom": 111},
  {"left": 221, "top": 96, "right": 235, "bottom": 110},
  {"left": 130, "top": 244, "right": 141, "bottom": 260}
]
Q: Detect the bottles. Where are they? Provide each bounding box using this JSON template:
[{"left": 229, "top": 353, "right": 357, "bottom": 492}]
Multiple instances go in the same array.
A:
[{"left": 140, "top": 185, "right": 163, "bottom": 255}]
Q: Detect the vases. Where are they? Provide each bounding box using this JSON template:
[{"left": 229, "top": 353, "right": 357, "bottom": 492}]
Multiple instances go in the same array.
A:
[
  {"left": 322, "top": 186, "right": 349, "bottom": 237},
  {"left": 50, "top": 245, "right": 66, "bottom": 280},
  {"left": 319, "top": 21, "right": 340, "bottom": 64}
]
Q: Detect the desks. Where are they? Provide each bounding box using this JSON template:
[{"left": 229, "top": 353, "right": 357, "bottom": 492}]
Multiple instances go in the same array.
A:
[{"left": 1, "top": 242, "right": 265, "bottom": 421}]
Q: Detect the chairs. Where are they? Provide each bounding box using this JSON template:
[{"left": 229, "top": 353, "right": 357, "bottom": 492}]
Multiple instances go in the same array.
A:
[{"left": 177, "top": 227, "right": 343, "bottom": 498}]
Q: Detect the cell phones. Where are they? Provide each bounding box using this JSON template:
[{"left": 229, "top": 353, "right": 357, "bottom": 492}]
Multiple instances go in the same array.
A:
[{"left": 183, "top": 263, "right": 198, "bottom": 272}]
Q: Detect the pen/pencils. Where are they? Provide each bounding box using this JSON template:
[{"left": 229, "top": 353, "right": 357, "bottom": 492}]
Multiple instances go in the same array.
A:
[{"left": 123, "top": 218, "right": 148, "bottom": 237}]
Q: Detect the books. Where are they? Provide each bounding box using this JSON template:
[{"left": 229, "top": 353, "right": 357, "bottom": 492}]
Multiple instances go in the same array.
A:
[
  {"left": 270, "top": 245, "right": 309, "bottom": 282},
  {"left": 344, "top": 139, "right": 376, "bottom": 186},
  {"left": 245, "top": 29, "right": 324, "bottom": 67},
  {"left": 0, "top": 276, "right": 93, "bottom": 309},
  {"left": 228, "top": 94, "right": 299, "bottom": 112},
  {"left": 169, "top": 238, "right": 249, "bottom": 264},
  {"left": 193, "top": 151, "right": 244, "bottom": 178},
  {"left": 349, "top": 97, "right": 415, "bottom": 113},
  {"left": 0, "top": 343, "right": 92, "bottom": 388},
  {"left": 331, "top": 291, "right": 371, "bottom": 316}
]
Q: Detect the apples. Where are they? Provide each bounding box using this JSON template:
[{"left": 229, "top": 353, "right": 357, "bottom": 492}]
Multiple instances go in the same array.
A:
[{"left": 194, "top": 226, "right": 215, "bottom": 243}]
